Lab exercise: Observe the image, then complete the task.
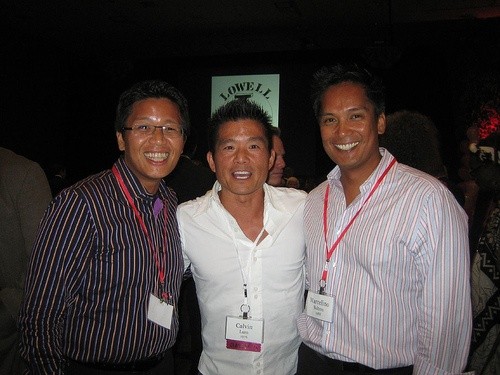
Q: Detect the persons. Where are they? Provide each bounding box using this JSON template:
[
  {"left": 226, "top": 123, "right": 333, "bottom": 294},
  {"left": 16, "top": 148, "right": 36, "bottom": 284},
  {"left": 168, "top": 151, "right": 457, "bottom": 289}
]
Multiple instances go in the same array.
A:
[
  {"left": 0, "top": 107, "right": 500, "bottom": 375},
  {"left": 174, "top": 99, "right": 324, "bottom": 375},
  {"left": 19, "top": 79, "right": 200, "bottom": 375},
  {"left": 298, "top": 62, "right": 472, "bottom": 375}
]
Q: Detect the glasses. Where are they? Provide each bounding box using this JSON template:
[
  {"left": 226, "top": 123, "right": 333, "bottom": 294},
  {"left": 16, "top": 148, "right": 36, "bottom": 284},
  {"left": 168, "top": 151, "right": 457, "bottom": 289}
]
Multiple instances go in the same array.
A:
[{"left": 120, "top": 120, "right": 185, "bottom": 141}]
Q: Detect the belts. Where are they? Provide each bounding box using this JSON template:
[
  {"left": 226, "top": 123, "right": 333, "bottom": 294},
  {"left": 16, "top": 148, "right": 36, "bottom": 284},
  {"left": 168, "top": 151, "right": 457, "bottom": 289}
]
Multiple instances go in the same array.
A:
[{"left": 71, "top": 357, "right": 170, "bottom": 372}]
